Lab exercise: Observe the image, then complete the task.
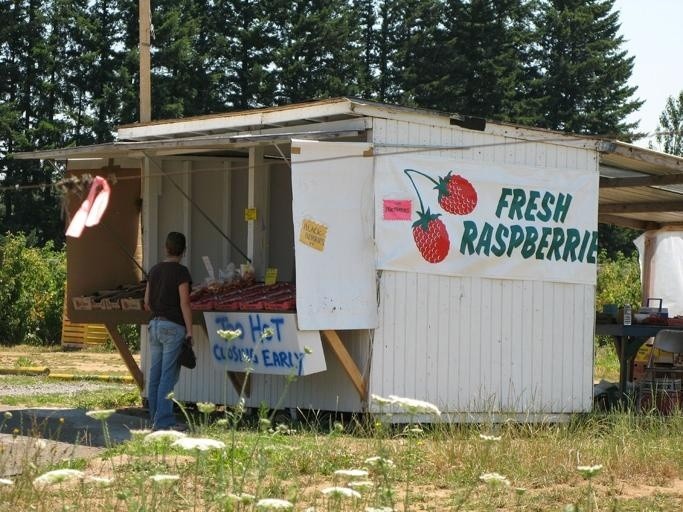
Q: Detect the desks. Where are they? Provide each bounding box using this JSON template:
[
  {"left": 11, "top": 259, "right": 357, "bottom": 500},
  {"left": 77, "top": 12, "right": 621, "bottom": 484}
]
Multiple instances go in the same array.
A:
[{"left": 597, "top": 322, "right": 682, "bottom": 413}]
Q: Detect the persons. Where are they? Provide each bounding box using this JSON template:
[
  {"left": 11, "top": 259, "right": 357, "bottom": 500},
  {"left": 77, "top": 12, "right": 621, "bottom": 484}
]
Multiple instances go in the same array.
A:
[{"left": 142, "top": 232, "right": 195, "bottom": 431}]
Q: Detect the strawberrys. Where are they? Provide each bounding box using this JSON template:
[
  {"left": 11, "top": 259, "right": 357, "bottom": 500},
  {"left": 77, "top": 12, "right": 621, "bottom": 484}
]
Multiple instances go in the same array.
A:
[
  {"left": 404, "top": 170, "right": 449, "bottom": 263},
  {"left": 405, "top": 169, "right": 477, "bottom": 215}
]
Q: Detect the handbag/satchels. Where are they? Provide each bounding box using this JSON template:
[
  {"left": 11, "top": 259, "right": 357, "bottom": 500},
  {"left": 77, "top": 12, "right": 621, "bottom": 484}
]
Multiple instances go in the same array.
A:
[{"left": 179, "top": 335, "right": 197, "bottom": 370}]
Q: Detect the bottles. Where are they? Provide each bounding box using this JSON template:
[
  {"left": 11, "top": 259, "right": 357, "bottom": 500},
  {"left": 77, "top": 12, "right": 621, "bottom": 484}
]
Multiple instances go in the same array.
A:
[{"left": 623, "top": 303, "right": 632, "bottom": 326}]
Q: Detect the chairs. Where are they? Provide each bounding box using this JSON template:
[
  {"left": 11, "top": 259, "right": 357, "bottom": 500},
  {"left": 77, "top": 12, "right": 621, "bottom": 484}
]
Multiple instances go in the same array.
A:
[{"left": 635, "top": 330, "right": 683, "bottom": 417}]
